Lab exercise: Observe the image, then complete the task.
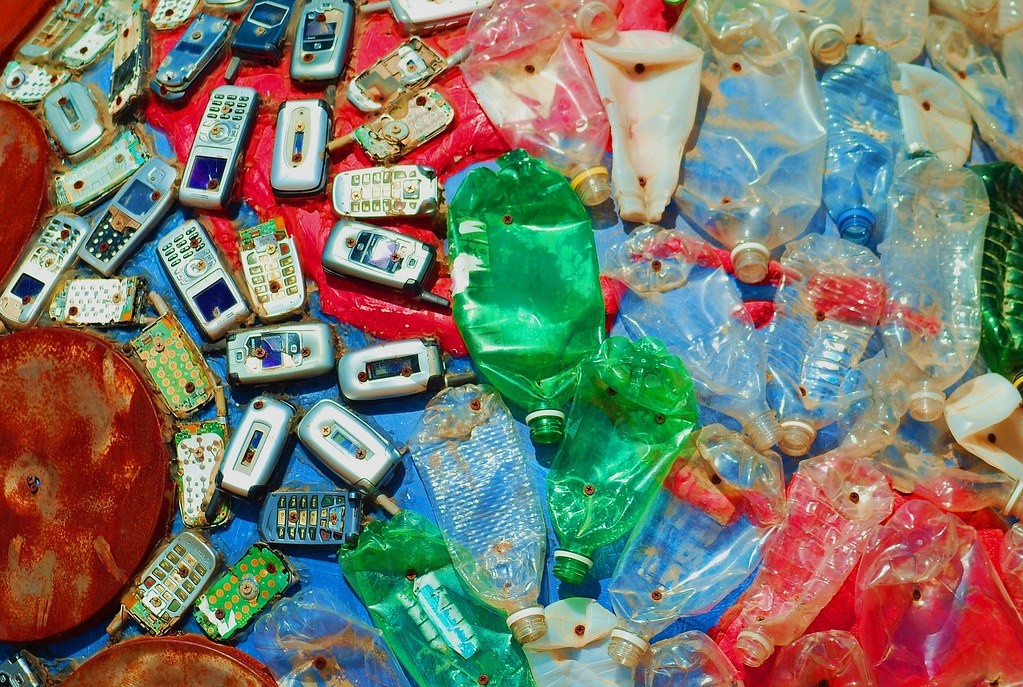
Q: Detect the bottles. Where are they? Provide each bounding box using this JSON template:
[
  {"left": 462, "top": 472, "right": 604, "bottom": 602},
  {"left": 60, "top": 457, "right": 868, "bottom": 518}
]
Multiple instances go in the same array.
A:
[
  {"left": 447, "top": 0, "right": 1023, "bottom": 284},
  {"left": 252, "top": 145, "right": 1023, "bottom": 687}
]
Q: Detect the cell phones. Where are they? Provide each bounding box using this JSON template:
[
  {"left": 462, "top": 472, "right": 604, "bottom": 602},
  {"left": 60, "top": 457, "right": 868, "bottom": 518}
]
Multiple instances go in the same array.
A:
[{"left": 0, "top": 0, "right": 497, "bottom": 642}]
